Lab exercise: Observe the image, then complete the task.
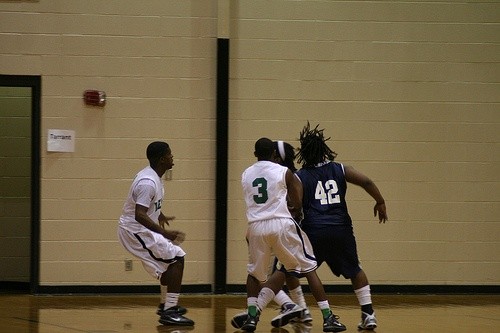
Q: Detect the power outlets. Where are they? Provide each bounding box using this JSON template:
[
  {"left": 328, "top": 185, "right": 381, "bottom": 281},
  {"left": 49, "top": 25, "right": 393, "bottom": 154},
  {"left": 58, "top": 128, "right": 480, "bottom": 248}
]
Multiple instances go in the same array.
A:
[{"left": 125, "top": 260, "right": 132, "bottom": 271}]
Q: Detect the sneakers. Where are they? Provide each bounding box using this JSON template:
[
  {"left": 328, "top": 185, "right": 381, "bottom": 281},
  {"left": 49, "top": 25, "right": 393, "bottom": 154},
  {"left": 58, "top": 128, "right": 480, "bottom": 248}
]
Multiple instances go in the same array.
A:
[
  {"left": 357, "top": 310, "right": 377, "bottom": 330},
  {"left": 159, "top": 304, "right": 195, "bottom": 326},
  {"left": 231, "top": 307, "right": 261, "bottom": 329},
  {"left": 323, "top": 310, "right": 346, "bottom": 331},
  {"left": 240, "top": 313, "right": 258, "bottom": 333},
  {"left": 156, "top": 303, "right": 188, "bottom": 315},
  {"left": 271, "top": 302, "right": 303, "bottom": 328},
  {"left": 289, "top": 309, "right": 313, "bottom": 323}
]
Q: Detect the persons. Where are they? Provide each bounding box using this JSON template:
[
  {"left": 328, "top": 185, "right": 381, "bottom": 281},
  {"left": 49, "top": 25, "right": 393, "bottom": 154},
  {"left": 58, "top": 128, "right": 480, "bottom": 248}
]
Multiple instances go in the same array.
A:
[
  {"left": 118, "top": 141, "right": 197, "bottom": 326},
  {"left": 239, "top": 137, "right": 348, "bottom": 333},
  {"left": 269, "top": 140, "right": 313, "bottom": 328},
  {"left": 231, "top": 117, "right": 390, "bottom": 330}
]
